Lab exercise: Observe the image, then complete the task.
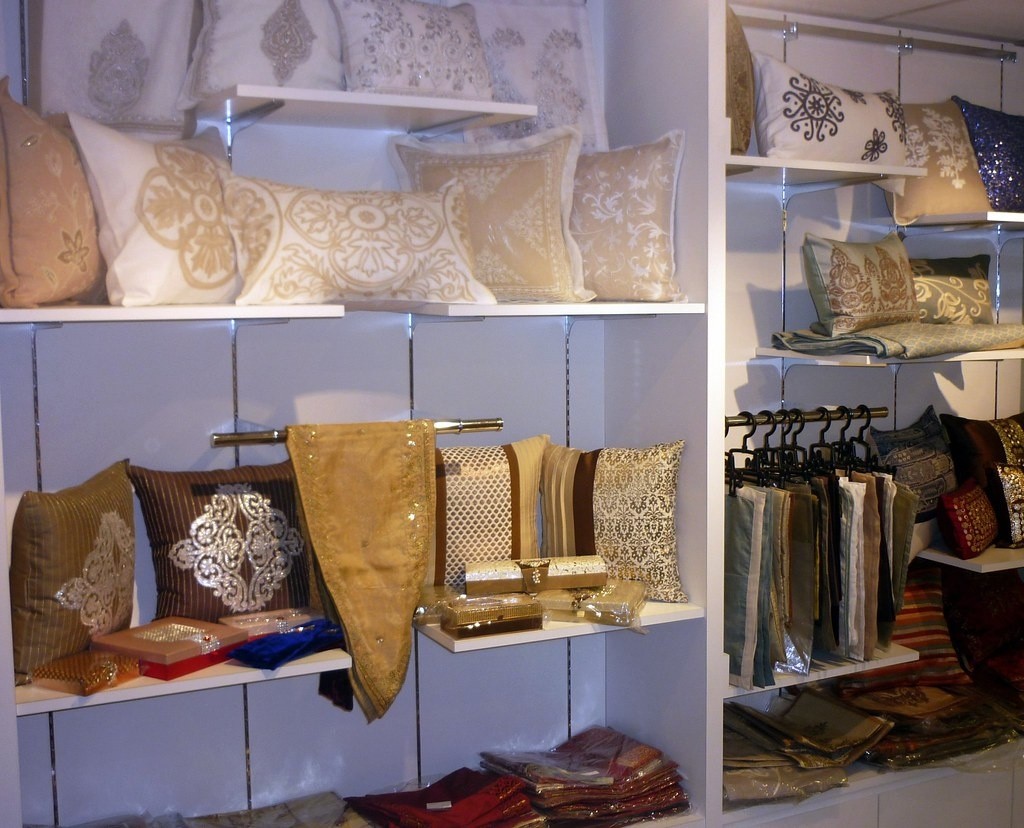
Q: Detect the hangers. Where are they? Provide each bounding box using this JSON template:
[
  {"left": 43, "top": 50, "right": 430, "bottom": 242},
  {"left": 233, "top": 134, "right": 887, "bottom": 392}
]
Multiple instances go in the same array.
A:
[{"left": 726, "top": 405, "right": 885, "bottom": 495}]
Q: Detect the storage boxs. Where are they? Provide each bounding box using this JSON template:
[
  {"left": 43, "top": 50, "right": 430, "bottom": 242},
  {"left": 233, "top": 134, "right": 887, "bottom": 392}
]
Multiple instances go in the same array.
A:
[
  {"left": 28, "top": 607, "right": 326, "bottom": 698},
  {"left": 416, "top": 555, "right": 648, "bottom": 640}
]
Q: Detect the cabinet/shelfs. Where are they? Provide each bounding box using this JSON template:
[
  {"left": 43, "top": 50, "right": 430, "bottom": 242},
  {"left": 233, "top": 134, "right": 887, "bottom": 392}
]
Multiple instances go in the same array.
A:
[
  {"left": 0, "top": 0, "right": 726, "bottom": 828},
  {"left": 725, "top": 0, "right": 1024, "bottom": 828},
  {"left": 723, "top": 735, "right": 1024, "bottom": 828}
]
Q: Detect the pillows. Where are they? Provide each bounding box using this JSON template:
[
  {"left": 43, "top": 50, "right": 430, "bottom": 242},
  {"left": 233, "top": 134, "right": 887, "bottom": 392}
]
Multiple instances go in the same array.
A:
[
  {"left": 863, "top": 405, "right": 957, "bottom": 522},
  {"left": 9, "top": 459, "right": 135, "bottom": 687},
  {"left": 726, "top": 3, "right": 755, "bottom": 157},
  {"left": 63, "top": 109, "right": 240, "bottom": 307},
  {"left": 382, "top": 126, "right": 597, "bottom": 303},
  {"left": 951, "top": 95, "right": 1024, "bottom": 214},
  {"left": 751, "top": 50, "right": 906, "bottom": 195},
  {"left": 178, "top": 0, "right": 345, "bottom": 110},
  {"left": 837, "top": 568, "right": 973, "bottom": 703},
  {"left": 885, "top": 100, "right": 996, "bottom": 227},
  {"left": 990, "top": 461, "right": 1024, "bottom": 550},
  {"left": 909, "top": 255, "right": 994, "bottom": 325},
  {"left": 217, "top": 166, "right": 498, "bottom": 307},
  {"left": 938, "top": 410, "right": 1023, "bottom": 486},
  {"left": 540, "top": 441, "right": 689, "bottom": 604},
  {"left": 441, "top": 0, "right": 597, "bottom": 153},
  {"left": 330, "top": 0, "right": 494, "bottom": 101},
  {"left": 800, "top": 230, "right": 920, "bottom": 337},
  {"left": 432, "top": 436, "right": 549, "bottom": 584},
  {"left": 956, "top": 568, "right": 1024, "bottom": 640},
  {"left": 28, "top": 0, "right": 200, "bottom": 133},
  {"left": 0, "top": 74, "right": 106, "bottom": 303},
  {"left": 939, "top": 476, "right": 997, "bottom": 560},
  {"left": 126, "top": 459, "right": 320, "bottom": 622},
  {"left": 576, "top": 129, "right": 687, "bottom": 305}
]
